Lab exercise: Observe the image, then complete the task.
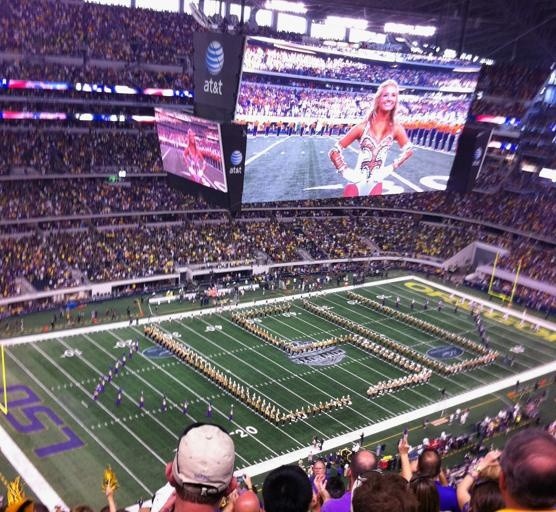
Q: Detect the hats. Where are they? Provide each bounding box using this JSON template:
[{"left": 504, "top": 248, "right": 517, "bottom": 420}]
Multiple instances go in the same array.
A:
[{"left": 171, "top": 423, "right": 236, "bottom": 496}]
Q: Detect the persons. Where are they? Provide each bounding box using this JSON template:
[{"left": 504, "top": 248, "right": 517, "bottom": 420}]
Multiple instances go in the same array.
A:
[{"left": 1, "top": 0, "right": 555, "bottom": 511}]
